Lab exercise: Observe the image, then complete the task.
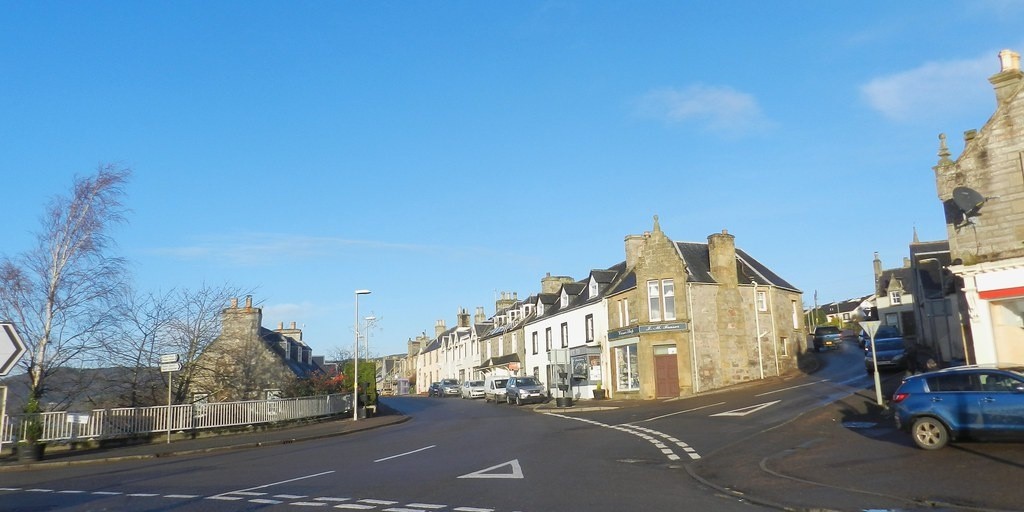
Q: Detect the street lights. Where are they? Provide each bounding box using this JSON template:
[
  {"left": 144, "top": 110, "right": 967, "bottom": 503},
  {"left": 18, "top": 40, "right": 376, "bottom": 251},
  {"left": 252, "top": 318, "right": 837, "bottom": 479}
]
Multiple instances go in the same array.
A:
[{"left": 354, "top": 290, "right": 376, "bottom": 420}]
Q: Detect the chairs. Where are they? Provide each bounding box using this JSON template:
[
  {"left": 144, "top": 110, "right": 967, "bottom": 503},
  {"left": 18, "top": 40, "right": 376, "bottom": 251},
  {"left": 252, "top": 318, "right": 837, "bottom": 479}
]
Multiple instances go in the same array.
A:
[{"left": 985, "top": 376, "right": 1008, "bottom": 391}]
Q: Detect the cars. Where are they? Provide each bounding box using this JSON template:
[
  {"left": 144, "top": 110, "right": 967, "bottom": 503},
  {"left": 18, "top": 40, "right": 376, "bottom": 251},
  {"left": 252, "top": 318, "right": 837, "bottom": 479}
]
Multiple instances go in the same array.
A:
[
  {"left": 462, "top": 380, "right": 484, "bottom": 399},
  {"left": 860, "top": 326, "right": 907, "bottom": 374},
  {"left": 379, "top": 388, "right": 393, "bottom": 396},
  {"left": 427, "top": 382, "right": 439, "bottom": 397}
]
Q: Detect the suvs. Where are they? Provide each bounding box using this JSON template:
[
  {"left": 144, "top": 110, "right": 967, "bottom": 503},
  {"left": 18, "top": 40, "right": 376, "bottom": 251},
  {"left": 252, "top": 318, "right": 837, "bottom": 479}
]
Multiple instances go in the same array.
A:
[
  {"left": 437, "top": 378, "right": 463, "bottom": 398},
  {"left": 889, "top": 366, "right": 1024, "bottom": 450},
  {"left": 506, "top": 377, "right": 545, "bottom": 405}
]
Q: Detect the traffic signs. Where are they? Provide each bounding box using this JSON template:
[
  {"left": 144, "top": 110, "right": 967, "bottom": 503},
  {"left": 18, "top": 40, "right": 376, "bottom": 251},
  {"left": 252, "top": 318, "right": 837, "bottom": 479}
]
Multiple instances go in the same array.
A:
[{"left": 160, "top": 354, "right": 183, "bottom": 372}]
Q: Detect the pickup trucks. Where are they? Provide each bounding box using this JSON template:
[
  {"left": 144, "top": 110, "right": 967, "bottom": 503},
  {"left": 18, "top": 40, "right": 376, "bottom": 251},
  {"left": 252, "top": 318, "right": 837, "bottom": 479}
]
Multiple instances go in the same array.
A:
[{"left": 809, "top": 323, "right": 843, "bottom": 353}]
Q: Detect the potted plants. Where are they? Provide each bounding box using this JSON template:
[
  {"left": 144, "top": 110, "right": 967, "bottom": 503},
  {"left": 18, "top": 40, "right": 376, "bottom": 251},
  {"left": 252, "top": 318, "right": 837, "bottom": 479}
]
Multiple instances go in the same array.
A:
[
  {"left": 16, "top": 421, "right": 47, "bottom": 465},
  {"left": 592, "top": 381, "right": 605, "bottom": 400}
]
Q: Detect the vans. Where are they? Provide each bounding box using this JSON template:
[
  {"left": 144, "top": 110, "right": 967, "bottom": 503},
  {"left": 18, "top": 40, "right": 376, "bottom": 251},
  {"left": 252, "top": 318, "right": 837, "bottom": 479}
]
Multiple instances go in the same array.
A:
[{"left": 484, "top": 377, "right": 511, "bottom": 403}]
